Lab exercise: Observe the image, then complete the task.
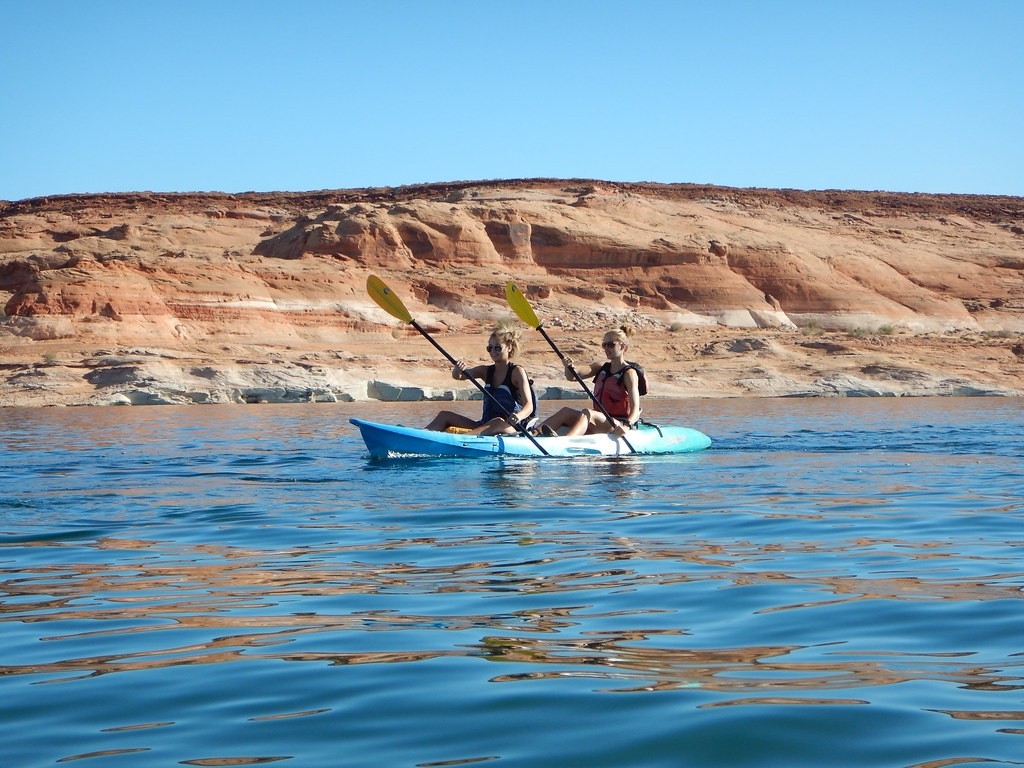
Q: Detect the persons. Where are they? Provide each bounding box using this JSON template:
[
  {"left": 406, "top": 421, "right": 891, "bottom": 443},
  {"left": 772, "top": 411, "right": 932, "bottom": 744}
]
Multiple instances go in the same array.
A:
[
  {"left": 424, "top": 330, "right": 533, "bottom": 436},
  {"left": 533, "top": 329, "right": 640, "bottom": 437}
]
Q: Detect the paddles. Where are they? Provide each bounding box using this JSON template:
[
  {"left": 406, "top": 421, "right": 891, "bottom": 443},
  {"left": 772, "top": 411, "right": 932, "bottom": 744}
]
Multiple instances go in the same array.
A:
[
  {"left": 366, "top": 275, "right": 551, "bottom": 458},
  {"left": 505, "top": 279, "right": 638, "bottom": 455}
]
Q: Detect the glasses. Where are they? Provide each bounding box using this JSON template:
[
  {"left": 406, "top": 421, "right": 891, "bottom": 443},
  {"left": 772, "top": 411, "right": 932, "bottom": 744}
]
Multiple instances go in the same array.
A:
[
  {"left": 602, "top": 342, "right": 622, "bottom": 348},
  {"left": 486, "top": 345, "right": 508, "bottom": 352}
]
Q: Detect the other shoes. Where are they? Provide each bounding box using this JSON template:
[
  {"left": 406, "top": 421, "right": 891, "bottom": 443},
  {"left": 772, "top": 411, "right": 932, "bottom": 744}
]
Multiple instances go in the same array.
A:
[{"left": 542, "top": 424, "right": 558, "bottom": 436}]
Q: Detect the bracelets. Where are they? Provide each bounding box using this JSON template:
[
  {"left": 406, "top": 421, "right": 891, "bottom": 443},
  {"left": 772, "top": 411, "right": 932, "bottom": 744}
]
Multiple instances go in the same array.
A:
[{"left": 623, "top": 419, "right": 633, "bottom": 429}]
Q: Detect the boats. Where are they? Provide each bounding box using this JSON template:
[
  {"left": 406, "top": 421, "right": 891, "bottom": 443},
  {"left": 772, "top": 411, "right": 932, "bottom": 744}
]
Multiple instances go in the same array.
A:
[{"left": 346, "top": 416, "right": 713, "bottom": 459}]
